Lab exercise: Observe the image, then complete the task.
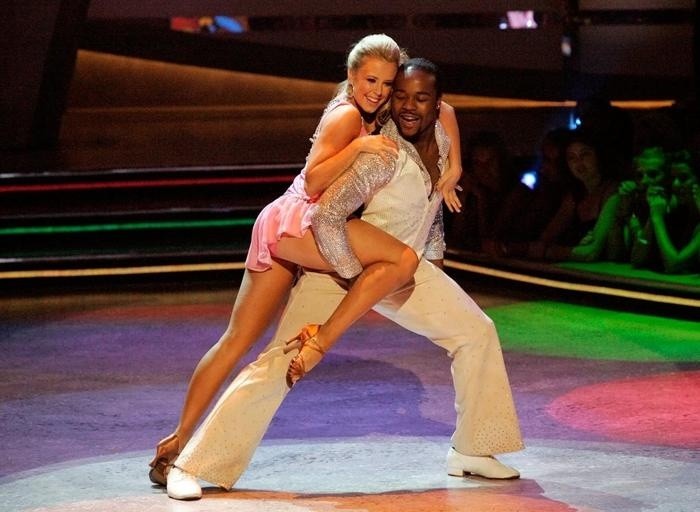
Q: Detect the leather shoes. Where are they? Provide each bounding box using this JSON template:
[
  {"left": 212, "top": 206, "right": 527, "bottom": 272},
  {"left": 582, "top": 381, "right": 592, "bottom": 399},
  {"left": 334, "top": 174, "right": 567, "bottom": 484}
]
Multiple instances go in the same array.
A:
[
  {"left": 167, "top": 464, "right": 202, "bottom": 499},
  {"left": 447, "top": 447, "right": 520, "bottom": 479}
]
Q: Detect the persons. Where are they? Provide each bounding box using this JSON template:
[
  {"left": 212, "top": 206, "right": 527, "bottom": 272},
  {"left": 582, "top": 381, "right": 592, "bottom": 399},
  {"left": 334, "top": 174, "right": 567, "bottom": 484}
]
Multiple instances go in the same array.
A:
[
  {"left": 167, "top": 58, "right": 524, "bottom": 502},
  {"left": 147, "top": 33, "right": 463, "bottom": 479},
  {"left": 443, "top": 73, "right": 700, "bottom": 277}
]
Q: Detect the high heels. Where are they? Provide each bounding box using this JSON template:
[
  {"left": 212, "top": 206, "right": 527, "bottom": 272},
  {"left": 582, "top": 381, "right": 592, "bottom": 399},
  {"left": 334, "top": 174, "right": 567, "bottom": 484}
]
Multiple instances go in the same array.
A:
[
  {"left": 149, "top": 435, "right": 179, "bottom": 486},
  {"left": 285, "top": 325, "right": 325, "bottom": 388}
]
